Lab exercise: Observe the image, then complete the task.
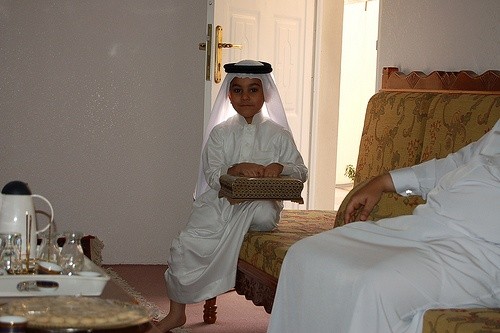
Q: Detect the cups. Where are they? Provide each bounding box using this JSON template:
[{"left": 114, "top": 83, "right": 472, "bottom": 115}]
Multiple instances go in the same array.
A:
[
  {"left": 0, "top": 233, "right": 22, "bottom": 274},
  {"left": 62, "top": 231, "right": 83, "bottom": 272},
  {"left": 38, "top": 231, "right": 59, "bottom": 274}
]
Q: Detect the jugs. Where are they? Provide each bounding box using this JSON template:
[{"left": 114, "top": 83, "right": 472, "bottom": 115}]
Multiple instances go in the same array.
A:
[{"left": 0, "top": 181, "right": 54, "bottom": 269}]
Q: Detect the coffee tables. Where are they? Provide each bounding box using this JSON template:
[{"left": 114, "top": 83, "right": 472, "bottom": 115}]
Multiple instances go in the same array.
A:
[{"left": 0, "top": 234, "right": 161, "bottom": 333}]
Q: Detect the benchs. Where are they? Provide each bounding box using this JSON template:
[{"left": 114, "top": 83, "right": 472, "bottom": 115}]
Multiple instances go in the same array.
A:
[{"left": 203, "top": 66, "right": 500, "bottom": 333}]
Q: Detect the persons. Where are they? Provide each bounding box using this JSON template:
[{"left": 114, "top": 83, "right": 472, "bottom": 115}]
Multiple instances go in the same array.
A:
[
  {"left": 265, "top": 119, "right": 500, "bottom": 332},
  {"left": 154, "top": 60, "right": 308, "bottom": 332}
]
